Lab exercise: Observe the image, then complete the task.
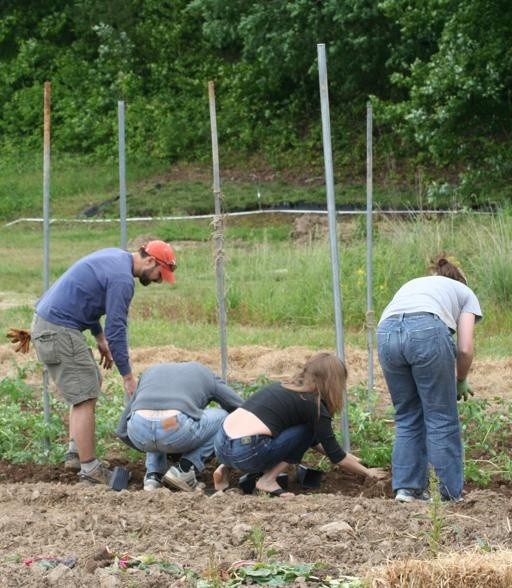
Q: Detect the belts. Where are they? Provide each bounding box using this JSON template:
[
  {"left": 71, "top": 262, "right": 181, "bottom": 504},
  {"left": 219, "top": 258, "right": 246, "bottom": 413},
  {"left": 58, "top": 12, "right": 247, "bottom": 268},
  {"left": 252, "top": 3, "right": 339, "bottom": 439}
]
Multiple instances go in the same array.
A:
[{"left": 391, "top": 312, "right": 431, "bottom": 318}]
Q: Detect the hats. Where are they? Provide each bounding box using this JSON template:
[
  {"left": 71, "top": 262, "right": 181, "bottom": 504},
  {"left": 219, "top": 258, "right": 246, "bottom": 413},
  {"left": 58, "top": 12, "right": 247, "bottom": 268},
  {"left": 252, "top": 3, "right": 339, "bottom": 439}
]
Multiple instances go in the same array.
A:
[{"left": 142, "top": 240, "right": 177, "bottom": 285}]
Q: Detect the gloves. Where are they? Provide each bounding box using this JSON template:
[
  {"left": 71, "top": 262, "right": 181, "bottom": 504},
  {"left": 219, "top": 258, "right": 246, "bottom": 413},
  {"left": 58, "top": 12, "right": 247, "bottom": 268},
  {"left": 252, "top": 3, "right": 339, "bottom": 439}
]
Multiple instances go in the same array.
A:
[{"left": 457, "top": 378, "right": 474, "bottom": 402}]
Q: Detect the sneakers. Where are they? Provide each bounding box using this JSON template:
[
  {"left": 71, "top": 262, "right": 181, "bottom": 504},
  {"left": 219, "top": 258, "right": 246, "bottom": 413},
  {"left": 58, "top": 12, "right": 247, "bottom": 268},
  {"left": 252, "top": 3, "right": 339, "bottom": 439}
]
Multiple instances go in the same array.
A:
[
  {"left": 64, "top": 452, "right": 112, "bottom": 484},
  {"left": 143, "top": 461, "right": 199, "bottom": 493},
  {"left": 395, "top": 488, "right": 430, "bottom": 503}
]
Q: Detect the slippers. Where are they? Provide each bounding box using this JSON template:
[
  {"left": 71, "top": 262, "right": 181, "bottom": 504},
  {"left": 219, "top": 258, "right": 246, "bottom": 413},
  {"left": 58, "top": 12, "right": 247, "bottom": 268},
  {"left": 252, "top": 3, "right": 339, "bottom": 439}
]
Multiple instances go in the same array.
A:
[{"left": 270, "top": 489, "right": 288, "bottom": 498}]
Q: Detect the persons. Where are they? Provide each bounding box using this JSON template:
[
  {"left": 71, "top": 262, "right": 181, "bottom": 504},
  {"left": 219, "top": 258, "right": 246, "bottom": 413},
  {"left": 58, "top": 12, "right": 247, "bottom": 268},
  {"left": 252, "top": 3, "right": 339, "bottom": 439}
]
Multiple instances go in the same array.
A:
[
  {"left": 117, "top": 361, "right": 246, "bottom": 492},
  {"left": 376, "top": 252, "right": 483, "bottom": 503},
  {"left": 31, "top": 241, "right": 176, "bottom": 484},
  {"left": 213, "top": 352, "right": 388, "bottom": 498}
]
